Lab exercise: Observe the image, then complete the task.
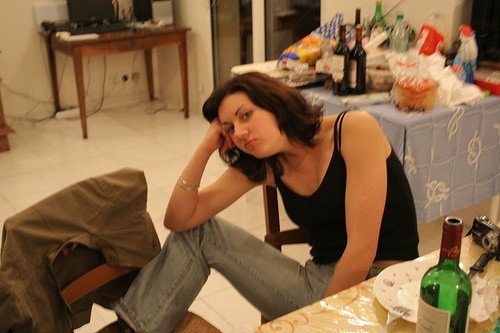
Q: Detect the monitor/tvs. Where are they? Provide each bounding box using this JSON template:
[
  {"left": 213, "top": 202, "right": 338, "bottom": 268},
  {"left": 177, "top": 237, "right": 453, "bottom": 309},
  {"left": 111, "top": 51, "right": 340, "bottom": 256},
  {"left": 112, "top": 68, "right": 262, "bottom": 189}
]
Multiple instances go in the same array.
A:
[{"left": 67, "top": 0, "right": 116, "bottom": 26}]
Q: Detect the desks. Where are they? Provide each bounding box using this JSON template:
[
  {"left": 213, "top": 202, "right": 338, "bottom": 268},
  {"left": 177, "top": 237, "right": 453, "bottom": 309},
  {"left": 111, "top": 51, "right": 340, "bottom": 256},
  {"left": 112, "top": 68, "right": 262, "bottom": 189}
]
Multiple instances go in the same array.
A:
[
  {"left": 45, "top": 21, "right": 192, "bottom": 139},
  {"left": 230, "top": 60, "right": 500, "bottom": 225}
]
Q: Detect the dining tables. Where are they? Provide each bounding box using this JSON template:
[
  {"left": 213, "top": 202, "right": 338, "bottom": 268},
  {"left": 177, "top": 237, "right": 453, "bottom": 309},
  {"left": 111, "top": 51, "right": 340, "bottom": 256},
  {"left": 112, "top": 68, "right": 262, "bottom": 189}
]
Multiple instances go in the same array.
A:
[{"left": 246, "top": 225, "right": 500, "bottom": 333}]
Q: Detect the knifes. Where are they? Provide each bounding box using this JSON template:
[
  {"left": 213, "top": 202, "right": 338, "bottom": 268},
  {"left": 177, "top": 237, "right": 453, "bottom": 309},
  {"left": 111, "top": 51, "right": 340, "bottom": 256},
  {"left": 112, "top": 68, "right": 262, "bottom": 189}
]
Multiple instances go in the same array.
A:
[{"left": 468, "top": 253, "right": 489, "bottom": 277}]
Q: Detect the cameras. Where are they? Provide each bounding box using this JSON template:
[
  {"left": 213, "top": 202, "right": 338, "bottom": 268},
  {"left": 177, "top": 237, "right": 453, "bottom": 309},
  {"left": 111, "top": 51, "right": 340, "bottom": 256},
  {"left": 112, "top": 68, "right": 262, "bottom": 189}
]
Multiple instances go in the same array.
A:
[{"left": 472, "top": 216, "right": 500, "bottom": 257}]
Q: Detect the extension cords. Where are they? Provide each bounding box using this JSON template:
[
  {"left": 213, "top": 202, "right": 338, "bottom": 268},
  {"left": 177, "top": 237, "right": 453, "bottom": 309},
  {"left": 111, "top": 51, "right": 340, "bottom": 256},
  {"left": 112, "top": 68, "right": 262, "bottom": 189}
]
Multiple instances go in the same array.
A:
[{"left": 54, "top": 108, "right": 80, "bottom": 121}]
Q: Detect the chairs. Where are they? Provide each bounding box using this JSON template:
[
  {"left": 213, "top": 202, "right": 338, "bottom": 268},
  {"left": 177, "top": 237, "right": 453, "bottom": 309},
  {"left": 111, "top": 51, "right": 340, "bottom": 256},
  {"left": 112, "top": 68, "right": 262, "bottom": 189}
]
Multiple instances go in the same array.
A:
[
  {"left": 261, "top": 183, "right": 308, "bottom": 326},
  {"left": 0, "top": 167, "right": 221, "bottom": 333}
]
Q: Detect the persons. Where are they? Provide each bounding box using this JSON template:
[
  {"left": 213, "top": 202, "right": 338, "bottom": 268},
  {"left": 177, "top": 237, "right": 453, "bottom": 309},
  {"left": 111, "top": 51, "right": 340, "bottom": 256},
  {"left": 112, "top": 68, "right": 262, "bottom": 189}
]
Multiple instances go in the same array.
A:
[{"left": 110, "top": 71, "right": 419, "bottom": 333}]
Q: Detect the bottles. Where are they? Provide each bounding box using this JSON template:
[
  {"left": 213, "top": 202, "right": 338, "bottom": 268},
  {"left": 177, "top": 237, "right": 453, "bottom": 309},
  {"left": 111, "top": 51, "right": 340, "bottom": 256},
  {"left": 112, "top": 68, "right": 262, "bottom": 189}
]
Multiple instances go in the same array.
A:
[
  {"left": 348, "top": 27, "right": 367, "bottom": 94},
  {"left": 352, "top": 9, "right": 364, "bottom": 38},
  {"left": 333, "top": 25, "right": 350, "bottom": 95},
  {"left": 416, "top": 216, "right": 472, "bottom": 333},
  {"left": 368, "top": 0, "right": 386, "bottom": 47},
  {"left": 389, "top": 15, "right": 409, "bottom": 53}
]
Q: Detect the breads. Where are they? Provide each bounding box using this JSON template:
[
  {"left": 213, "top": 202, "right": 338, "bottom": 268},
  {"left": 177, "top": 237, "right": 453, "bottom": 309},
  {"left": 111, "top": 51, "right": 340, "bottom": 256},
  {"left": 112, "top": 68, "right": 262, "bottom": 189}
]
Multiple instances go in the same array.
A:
[{"left": 394, "top": 79, "right": 440, "bottom": 112}]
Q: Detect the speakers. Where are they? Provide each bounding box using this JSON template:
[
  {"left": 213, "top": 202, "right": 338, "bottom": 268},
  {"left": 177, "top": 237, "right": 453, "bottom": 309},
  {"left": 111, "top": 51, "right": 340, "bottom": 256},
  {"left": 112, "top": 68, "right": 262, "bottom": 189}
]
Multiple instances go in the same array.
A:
[{"left": 133, "top": 0, "right": 152, "bottom": 22}]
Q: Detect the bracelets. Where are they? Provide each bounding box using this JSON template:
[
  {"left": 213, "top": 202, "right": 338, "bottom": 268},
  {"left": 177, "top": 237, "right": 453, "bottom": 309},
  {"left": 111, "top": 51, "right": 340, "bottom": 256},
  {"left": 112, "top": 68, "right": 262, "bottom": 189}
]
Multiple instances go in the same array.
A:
[{"left": 176, "top": 176, "right": 200, "bottom": 192}]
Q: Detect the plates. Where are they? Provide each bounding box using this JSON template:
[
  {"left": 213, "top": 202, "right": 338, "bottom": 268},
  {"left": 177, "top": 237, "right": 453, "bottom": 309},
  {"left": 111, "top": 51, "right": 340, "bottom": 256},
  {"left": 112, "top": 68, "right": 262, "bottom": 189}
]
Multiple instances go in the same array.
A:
[{"left": 373, "top": 258, "right": 478, "bottom": 324}]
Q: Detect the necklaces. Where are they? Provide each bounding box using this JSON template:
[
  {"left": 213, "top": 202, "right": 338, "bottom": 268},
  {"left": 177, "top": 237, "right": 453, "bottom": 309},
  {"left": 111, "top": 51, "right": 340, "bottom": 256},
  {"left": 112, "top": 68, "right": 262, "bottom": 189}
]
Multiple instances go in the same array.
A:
[{"left": 284, "top": 150, "right": 310, "bottom": 171}]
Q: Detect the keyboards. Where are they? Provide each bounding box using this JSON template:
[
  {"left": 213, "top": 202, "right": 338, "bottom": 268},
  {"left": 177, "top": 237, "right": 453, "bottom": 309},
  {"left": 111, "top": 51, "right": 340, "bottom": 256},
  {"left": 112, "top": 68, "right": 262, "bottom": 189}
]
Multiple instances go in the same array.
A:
[{"left": 70, "top": 24, "right": 129, "bottom": 35}]
